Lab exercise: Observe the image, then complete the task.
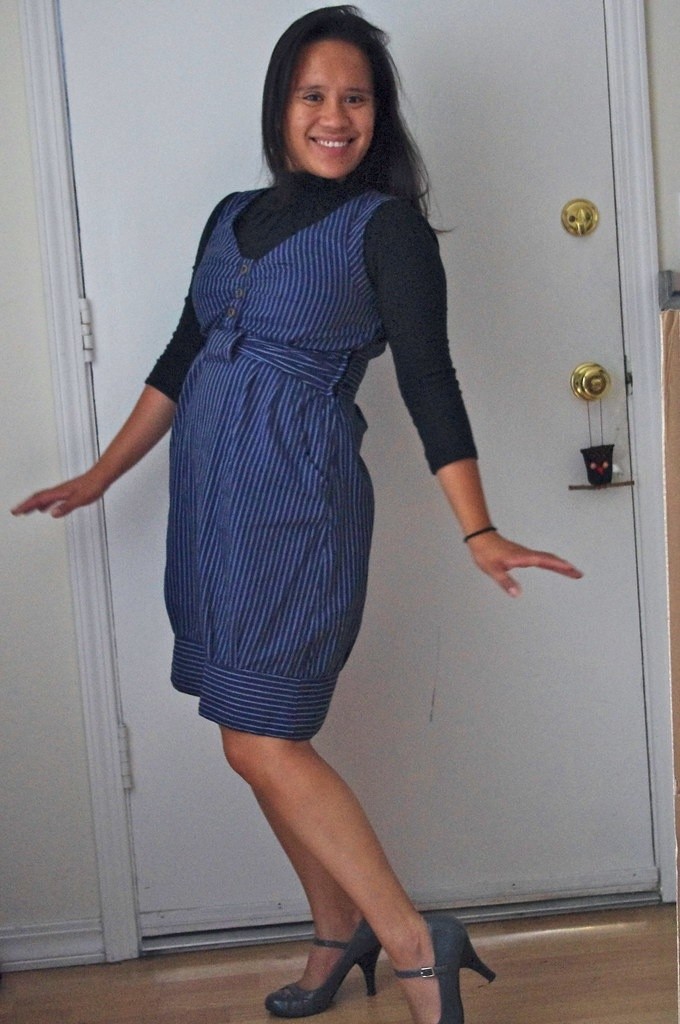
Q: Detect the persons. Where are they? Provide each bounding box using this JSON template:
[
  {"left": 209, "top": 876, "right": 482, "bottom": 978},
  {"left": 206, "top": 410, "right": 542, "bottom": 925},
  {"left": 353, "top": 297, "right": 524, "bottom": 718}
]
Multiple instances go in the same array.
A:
[{"left": 8, "top": 3, "right": 585, "bottom": 1024}]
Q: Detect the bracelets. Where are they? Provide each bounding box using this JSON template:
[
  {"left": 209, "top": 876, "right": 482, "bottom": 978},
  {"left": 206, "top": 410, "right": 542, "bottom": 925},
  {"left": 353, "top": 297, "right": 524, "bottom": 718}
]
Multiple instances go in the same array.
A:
[{"left": 462, "top": 527, "right": 498, "bottom": 542}]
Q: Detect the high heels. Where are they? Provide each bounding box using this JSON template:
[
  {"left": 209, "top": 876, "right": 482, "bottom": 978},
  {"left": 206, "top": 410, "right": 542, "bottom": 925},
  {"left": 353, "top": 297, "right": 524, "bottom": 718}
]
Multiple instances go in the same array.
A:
[
  {"left": 393, "top": 911, "right": 497, "bottom": 1024},
  {"left": 264, "top": 912, "right": 381, "bottom": 1019}
]
